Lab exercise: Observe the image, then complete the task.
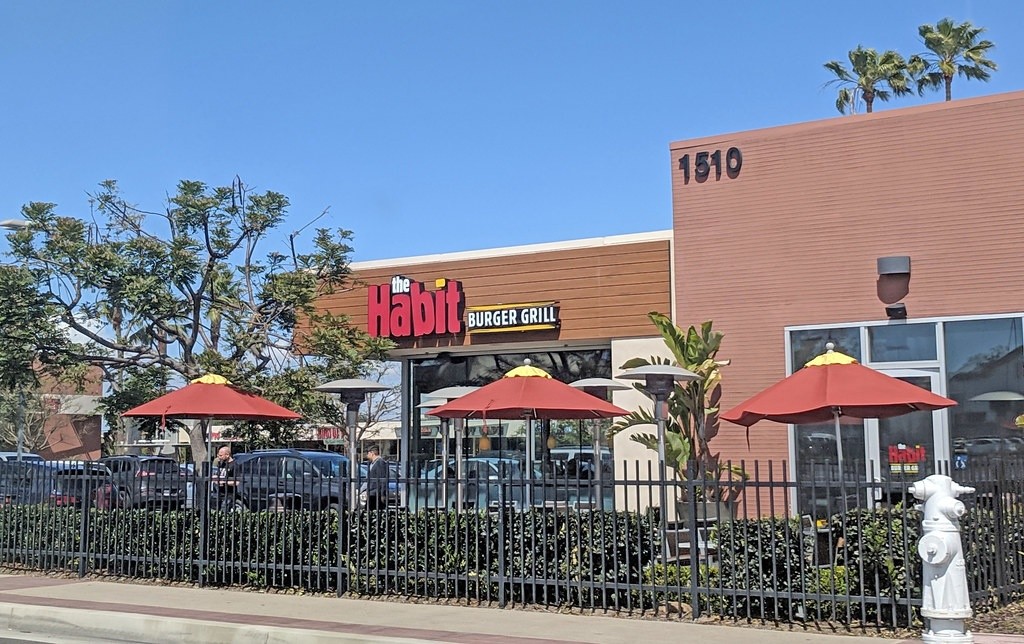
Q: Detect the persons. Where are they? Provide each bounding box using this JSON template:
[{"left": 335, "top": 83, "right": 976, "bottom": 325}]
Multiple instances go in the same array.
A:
[
  {"left": 213, "top": 445, "right": 240, "bottom": 511},
  {"left": 357, "top": 444, "right": 389, "bottom": 509}
]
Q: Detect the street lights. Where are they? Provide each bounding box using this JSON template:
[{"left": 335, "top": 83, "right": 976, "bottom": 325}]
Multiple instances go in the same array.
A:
[
  {"left": 615, "top": 364, "right": 706, "bottom": 530},
  {"left": 423, "top": 386, "right": 486, "bottom": 515},
  {"left": 312, "top": 379, "right": 395, "bottom": 513},
  {"left": 414, "top": 399, "right": 447, "bottom": 508},
  {"left": 565, "top": 377, "right": 634, "bottom": 515}
]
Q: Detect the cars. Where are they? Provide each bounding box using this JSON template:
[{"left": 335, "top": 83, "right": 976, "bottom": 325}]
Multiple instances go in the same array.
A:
[
  {"left": 358, "top": 443, "right": 615, "bottom": 518},
  {"left": 0, "top": 452, "right": 218, "bottom": 515},
  {"left": 803, "top": 429, "right": 1024, "bottom": 473}
]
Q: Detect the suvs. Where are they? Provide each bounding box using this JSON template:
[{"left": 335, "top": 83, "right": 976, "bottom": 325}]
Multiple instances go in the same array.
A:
[{"left": 209, "top": 446, "right": 369, "bottom": 515}]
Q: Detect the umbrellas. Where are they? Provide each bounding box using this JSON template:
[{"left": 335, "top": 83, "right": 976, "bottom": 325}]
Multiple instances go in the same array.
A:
[
  {"left": 123, "top": 374, "right": 304, "bottom": 508},
  {"left": 720, "top": 344, "right": 959, "bottom": 510},
  {"left": 425, "top": 358, "right": 632, "bottom": 510}
]
